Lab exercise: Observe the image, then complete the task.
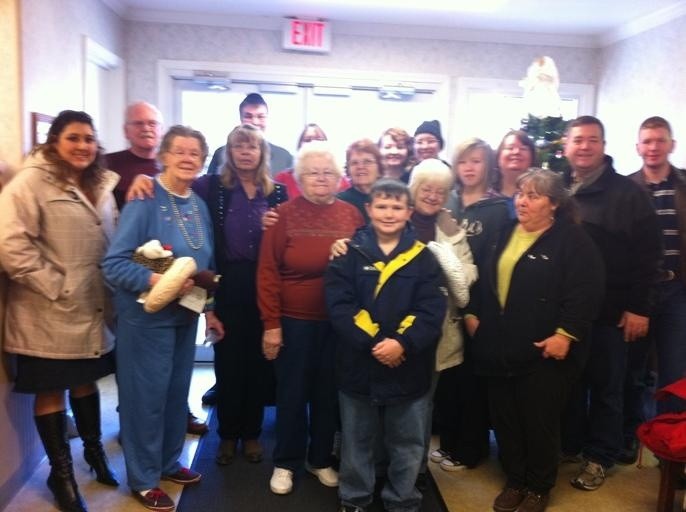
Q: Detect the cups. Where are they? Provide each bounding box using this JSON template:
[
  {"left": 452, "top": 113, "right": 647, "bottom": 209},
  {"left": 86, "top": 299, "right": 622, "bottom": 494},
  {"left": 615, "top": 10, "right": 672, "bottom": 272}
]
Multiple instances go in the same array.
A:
[{"left": 201, "top": 327, "right": 220, "bottom": 349}]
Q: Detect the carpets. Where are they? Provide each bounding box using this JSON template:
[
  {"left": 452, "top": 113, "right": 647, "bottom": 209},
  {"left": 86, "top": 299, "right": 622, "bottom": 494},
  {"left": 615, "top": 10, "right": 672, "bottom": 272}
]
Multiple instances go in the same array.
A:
[{"left": 173, "top": 400, "right": 450, "bottom": 512}]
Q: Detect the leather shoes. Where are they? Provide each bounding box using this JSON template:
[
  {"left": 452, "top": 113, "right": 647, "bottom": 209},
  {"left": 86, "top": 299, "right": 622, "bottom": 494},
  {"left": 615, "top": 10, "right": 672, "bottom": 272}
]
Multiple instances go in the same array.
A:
[
  {"left": 216, "top": 438, "right": 238, "bottom": 465},
  {"left": 241, "top": 438, "right": 264, "bottom": 462}
]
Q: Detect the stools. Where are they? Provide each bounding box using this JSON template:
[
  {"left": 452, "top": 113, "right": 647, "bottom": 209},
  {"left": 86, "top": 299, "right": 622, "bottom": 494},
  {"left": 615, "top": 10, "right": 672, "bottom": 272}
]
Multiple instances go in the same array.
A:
[{"left": 639, "top": 418, "right": 685, "bottom": 512}]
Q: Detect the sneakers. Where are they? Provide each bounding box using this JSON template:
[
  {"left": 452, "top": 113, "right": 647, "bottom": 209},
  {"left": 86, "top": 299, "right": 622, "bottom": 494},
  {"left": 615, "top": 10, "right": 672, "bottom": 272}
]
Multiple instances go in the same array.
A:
[
  {"left": 202, "top": 384, "right": 217, "bottom": 404},
  {"left": 269, "top": 466, "right": 294, "bottom": 495},
  {"left": 130, "top": 487, "right": 175, "bottom": 512},
  {"left": 431, "top": 448, "right": 470, "bottom": 471},
  {"left": 187, "top": 413, "right": 208, "bottom": 435},
  {"left": 161, "top": 465, "right": 201, "bottom": 485},
  {"left": 575, "top": 460, "right": 606, "bottom": 491},
  {"left": 493, "top": 487, "right": 548, "bottom": 511},
  {"left": 304, "top": 459, "right": 340, "bottom": 487}
]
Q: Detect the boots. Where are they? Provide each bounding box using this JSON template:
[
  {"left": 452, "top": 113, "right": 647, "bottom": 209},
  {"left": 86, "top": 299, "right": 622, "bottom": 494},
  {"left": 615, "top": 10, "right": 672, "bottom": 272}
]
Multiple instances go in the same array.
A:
[
  {"left": 68, "top": 391, "right": 120, "bottom": 486},
  {"left": 33, "top": 408, "right": 88, "bottom": 512}
]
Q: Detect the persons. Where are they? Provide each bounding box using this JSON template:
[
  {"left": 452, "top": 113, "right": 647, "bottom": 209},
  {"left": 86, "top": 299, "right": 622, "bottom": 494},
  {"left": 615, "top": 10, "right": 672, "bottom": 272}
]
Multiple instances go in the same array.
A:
[{"left": 0, "top": 92, "right": 685, "bottom": 510}]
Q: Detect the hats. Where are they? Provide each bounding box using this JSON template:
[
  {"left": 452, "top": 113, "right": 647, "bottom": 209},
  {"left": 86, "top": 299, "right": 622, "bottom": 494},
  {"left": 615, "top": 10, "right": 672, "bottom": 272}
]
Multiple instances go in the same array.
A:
[{"left": 414, "top": 120, "right": 443, "bottom": 150}]
